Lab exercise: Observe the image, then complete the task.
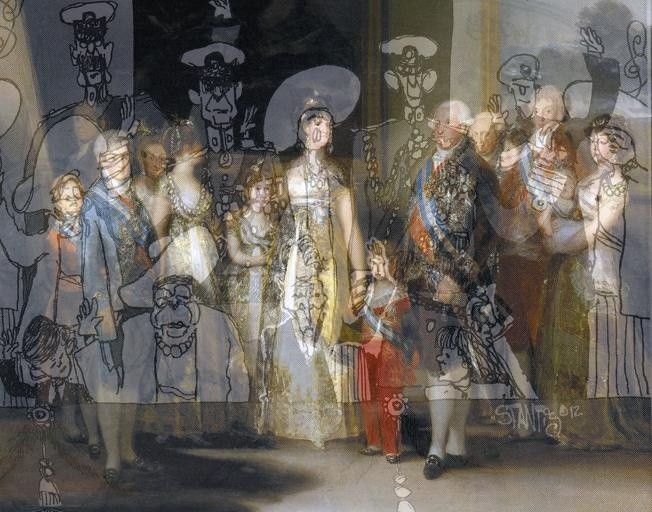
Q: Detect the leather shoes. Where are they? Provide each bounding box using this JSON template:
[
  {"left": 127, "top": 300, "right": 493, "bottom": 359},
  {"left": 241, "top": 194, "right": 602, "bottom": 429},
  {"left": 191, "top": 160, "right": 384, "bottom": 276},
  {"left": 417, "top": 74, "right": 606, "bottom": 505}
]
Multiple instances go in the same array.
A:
[
  {"left": 65, "top": 428, "right": 274, "bottom": 488},
  {"left": 360, "top": 421, "right": 518, "bottom": 480}
]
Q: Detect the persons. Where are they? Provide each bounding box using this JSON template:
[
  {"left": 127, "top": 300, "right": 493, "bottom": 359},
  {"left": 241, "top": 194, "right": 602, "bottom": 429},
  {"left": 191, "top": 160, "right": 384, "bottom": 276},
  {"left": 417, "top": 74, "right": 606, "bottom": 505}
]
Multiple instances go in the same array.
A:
[{"left": 43, "top": 83, "right": 652, "bottom": 489}]
